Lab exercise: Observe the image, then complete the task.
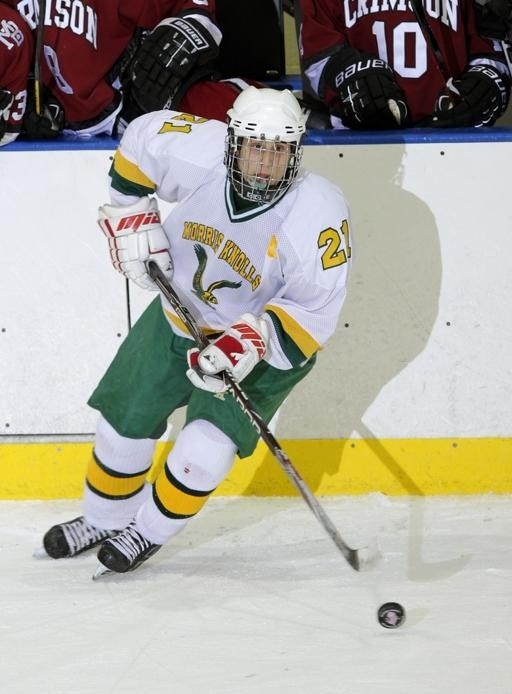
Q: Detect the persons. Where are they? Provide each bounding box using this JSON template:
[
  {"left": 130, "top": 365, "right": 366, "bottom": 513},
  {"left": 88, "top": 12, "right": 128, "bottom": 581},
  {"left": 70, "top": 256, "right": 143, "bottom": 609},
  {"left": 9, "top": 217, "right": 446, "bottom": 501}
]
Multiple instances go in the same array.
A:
[
  {"left": 40, "top": 73, "right": 356, "bottom": 574},
  {"left": 278, "top": 0, "right": 511, "bottom": 136},
  {"left": 7, "top": 0, "right": 331, "bottom": 143},
  {"left": 1, "top": 0, "right": 35, "bottom": 150}
]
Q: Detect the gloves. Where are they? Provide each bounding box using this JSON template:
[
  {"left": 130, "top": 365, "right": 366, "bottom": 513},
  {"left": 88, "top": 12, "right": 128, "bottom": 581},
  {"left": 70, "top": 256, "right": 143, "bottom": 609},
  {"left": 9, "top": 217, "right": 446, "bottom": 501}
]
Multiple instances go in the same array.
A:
[
  {"left": 429, "top": 64, "right": 509, "bottom": 128},
  {"left": 334, "top": 53, "right": 409, "bottom": 129},
  {"left": 185, "top": 314, "right": 270, "bottom": 394},
  {"left": 99, "top": 194, "right": 175, "bottom": 290},
  {"left": 127, "top": 16, "right": 219, "bottom": 101}
]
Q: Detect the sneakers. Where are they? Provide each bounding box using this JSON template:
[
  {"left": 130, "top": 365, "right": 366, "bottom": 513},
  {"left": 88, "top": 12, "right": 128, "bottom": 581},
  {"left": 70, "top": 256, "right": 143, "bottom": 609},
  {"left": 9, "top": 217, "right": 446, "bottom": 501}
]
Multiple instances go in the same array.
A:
[
  {"left": 97, "top": 518, "right": 162, "bottom": 573},
  {"left": 44, "top": 516, "right": 115, "bottom": 559}
]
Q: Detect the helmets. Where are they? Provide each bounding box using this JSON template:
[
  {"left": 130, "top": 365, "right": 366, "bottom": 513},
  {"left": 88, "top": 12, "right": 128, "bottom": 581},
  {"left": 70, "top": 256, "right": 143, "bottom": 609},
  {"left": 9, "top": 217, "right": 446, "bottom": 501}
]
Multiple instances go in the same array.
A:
[{"left": 222, "top": 85, "right": 307, "bottom": 162}]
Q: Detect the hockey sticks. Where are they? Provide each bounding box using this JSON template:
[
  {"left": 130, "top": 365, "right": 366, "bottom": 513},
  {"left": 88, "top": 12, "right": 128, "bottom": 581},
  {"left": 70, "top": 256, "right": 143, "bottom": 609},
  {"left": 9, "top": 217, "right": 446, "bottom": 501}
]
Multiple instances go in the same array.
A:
[{"left": 143, "top": 258, "right": 391, "bottom": 571}]
